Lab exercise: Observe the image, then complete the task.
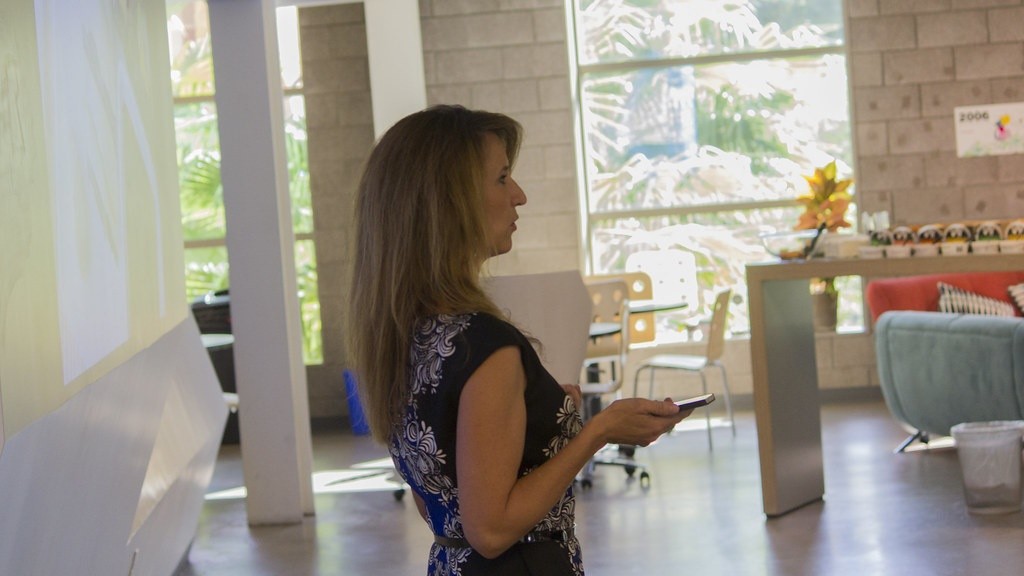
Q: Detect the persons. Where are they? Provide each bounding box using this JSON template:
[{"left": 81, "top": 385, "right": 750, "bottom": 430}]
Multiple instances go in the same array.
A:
[{"left": 347, "top": 103, "right": 697, "bottom": 575}]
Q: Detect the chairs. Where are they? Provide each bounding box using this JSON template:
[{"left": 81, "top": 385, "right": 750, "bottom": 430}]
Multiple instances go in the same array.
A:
[
  {"left": 577, "top": 278, "right": 631, "bottom": 402},
  {"left": 624, "top": 289, "right": 737, "bottom": 455},
  {"left": 574, "top": 297, "right": 633, "bottom": 426},
  {"left": 581, "top": 271, "right": 655, "bottom": 364}
]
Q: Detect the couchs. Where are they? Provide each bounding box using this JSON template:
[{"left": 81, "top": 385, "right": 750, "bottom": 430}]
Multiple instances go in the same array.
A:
[
  {"left": 870, "top": 305, "right": 1023, "bottom": 454},
  {"left": 865, "top": 271, "right": 1024, "bottom": 330}
]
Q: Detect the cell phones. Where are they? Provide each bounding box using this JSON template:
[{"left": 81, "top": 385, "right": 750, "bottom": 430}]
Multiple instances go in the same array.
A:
[{"left": 673, "top": 393, "right": 714, "bottom": 412}]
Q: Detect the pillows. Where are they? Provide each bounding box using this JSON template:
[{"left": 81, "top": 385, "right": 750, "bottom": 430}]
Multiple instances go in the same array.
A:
[
  {"left": 1003, "top": 278, "right": 1023, "bottom": 316},
  {"left": 934, "top": 280, "right": 1015, "bottom": 322}
]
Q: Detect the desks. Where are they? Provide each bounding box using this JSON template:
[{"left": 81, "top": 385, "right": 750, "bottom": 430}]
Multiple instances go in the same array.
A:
[
  {"left": 567, "top": 321, "right": 653, "bottom": 491},
  {"left": 620, "top": 296, "right": 689, "bottom": 320},
  {"left": 742, "top": 250, "right": 1024, "bottom": 517}
]
